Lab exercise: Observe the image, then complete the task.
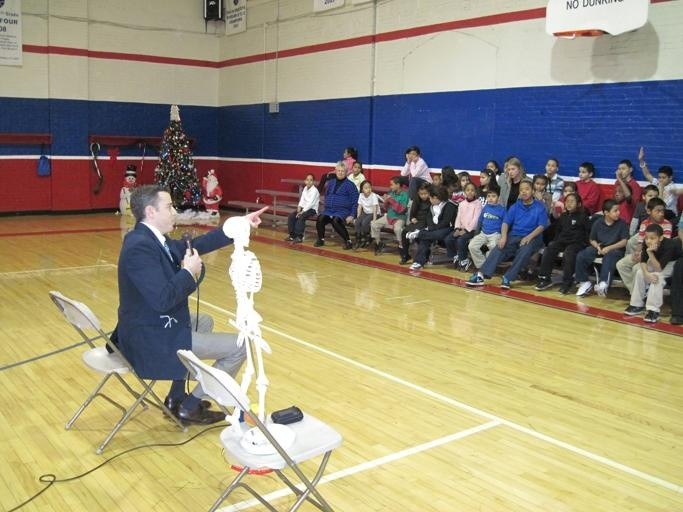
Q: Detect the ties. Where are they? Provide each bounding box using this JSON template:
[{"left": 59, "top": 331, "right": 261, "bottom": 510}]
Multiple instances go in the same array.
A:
[{"left": 164, "top": 242, "right": 174, "bottom": 263}]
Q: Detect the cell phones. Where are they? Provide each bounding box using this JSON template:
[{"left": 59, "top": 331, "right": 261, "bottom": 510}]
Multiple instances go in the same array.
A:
[{"left": 271, "top": 405, "right": 303, "bottom": 425}]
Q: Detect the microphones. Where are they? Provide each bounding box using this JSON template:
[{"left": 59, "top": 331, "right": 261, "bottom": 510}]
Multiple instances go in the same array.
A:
[{"left": 182, "top": 232, "right": 194, "bottom": 256}]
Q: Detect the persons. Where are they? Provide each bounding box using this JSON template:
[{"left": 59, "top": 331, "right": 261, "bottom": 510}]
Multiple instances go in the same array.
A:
[
  {"left": 202, "top": 166, "right": 222, "bottom": 211},
  {"left": 370, "top": 146, "right": 642, "bottom": 298},
  {"left": 314, "top": 146, "right": 381, "bottom": 251},
  {"left": 114, "top": 185, "right": 270, "bottom": 424},
  {"left": 615, "top": 147, "right": 682, "bottom": 325},
  {"left": 283, "top": 172, "right": 319, "bottom": 244}
]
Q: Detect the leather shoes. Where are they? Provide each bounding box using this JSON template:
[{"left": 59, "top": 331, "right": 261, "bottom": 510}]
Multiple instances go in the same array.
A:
[{"left": 163, "top": 393, "right": 225, "bottom": 426}]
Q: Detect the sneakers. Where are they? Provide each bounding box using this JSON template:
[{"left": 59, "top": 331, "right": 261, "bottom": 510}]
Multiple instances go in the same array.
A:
[
  {"left": 399, "top": 248, "right": 410, "bottom": 264},
  {"left": 313, "top": 239, "right": 325, "bottom": 246},
  {"left": 644, "top": 310, "right": 660, "bottom": 322},
  {"left": 343, "top": 240, "right": 384, "bottom": 256},
  {"left": 535, "top": 275, "right": 608, "bottom": 297},
  {"left": 671, "top": 315, "right": 683, "bottom": 324},
  {"left": 410, "top": 262, "right": 424, "bottom": 269},
  {"left": 284, "top": 235, "right": 303, "bottom": 242},
  {"left": 501, "top": 275, "right": 510, "bottom": 289},
  {"left": 624, "top": 305, "right": 644, "bottom": 315},
  {"left": 406, "top": 229, "right": 420, "bottom": 239},
  {"left": 447, "top": 255, "right": 472, "bottom": 271},
  {"left": 465, "top": 273, "right": 484, "bottom": 285}
]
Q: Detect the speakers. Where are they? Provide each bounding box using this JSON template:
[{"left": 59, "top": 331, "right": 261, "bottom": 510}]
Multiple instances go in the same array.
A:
[{"left": 203, "top": 0, "right": 222, "bottom": 21}]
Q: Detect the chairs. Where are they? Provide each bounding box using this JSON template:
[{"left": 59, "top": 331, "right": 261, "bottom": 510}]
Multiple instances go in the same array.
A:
[
  {"left": 49, "top": 290, "right": 188, "bottom": 455},
  {"left": 176, "top": 349, "right": 341, "bottom": 512}
]
